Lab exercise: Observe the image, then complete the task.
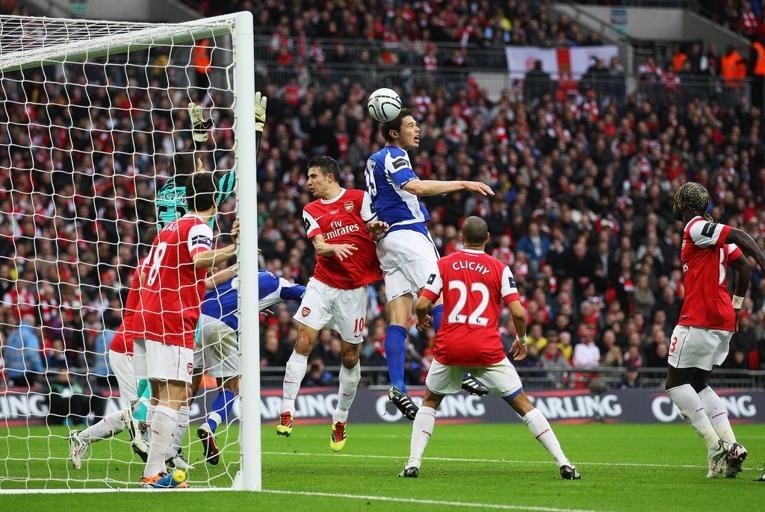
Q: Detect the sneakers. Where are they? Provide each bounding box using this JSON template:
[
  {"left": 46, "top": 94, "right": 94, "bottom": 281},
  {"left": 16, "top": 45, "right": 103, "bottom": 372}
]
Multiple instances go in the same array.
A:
[
  {"left": 560, "top": 465, "right": 580, "bottom": 479},
  {"left": 462, "top": 376, "right": 490, "bottom": 396},
  {"left": 388, "top": 386, "right": 419, "bottom": 420},
  {"left": 329, "top": 421, "right": 347, "bottom": 451},
  {"left": 706, "top": 439, "right": 728, "bottom": 477},
  {"left": 725, "top": 443, "right": 747, "bottom": 477},
  {"left": 139, "top": 472, "right": 187, "bottom": 488},
  {"left": 132, "top": 440, "right": 149, "bottom": 463},
  {"left": 166, "top": 456, "right": 194, "bottom": 469},
  {"left": 197, "top": 423, "right": 219, "bottom": 464},
  {"left": 276, "top": 410, "right": 293, "bottom": 435},
  {"left": 398, "top": 465, "right": 419, "bottom": 477},
  {"left": 68, "top": 430, "right": 88, "bottom": 469}
]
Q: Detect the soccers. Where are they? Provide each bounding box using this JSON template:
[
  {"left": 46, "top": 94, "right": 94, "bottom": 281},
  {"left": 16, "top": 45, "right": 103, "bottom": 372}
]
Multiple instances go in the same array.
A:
[{"left": 367, "top": 88, "right": 401, "bottom": 121}]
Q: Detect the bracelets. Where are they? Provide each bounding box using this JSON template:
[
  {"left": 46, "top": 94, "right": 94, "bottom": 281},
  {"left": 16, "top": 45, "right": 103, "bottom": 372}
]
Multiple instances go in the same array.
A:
[{"left": 516, "top": 332, "right": 526, "bottom": 344}]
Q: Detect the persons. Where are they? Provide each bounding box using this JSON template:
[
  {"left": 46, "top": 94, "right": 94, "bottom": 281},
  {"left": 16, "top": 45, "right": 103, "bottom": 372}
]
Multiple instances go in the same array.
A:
[
  {"left": 3, "top": 183, "right": 305, "bottom": 254},
  {"left": 496, "top": 182, "right": 765, "bottom": 216},
  {"left": 262, "top": 301, "right": 435, "bottom": 387},
  {"left": 0, "top": 256, "right": 129, "bottom": 426},
  {"left": 140, "top": 173, "right": 239, "bottom": 489},
  {"left": 133, "top": 91, "right": 266, "bottom": 466},
  {"left": 129, "top": 252, "right": 305, "bottom": 466},
  {"left": 397, "top": 216, "right": 581, "bottom": 479},
  {"left": 582, "top": 215, "right": 680, "bottom": 366},
  {"left": 365, "top": 112, "right": 495, "bottom": 422},
  {"left": 495, "top": 218, "right": 578, "bottom": 366},
  {"left": 0, "top": 2, "right": 765, "bottom": 181},
  {"left": 726, "top": 217, "right": 765, "bottom": 370},
  {"left": 664, "top": 181, "right": 765, "bottom": 479},
  {"left": 274, "top": 157, "right": 389, "bottom": 452},
  {"left": 68, "top": 256, "right": 241, "bottom": 468}
]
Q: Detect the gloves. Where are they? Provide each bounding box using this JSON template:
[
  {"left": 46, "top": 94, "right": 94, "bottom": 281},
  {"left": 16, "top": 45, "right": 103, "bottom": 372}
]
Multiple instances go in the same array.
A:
[
  {"left": 255, "top": 91, "right": 267, "bottom": 131},
  {"left": 188, "top": 103, "right": 214, "bottom": 142}
]
